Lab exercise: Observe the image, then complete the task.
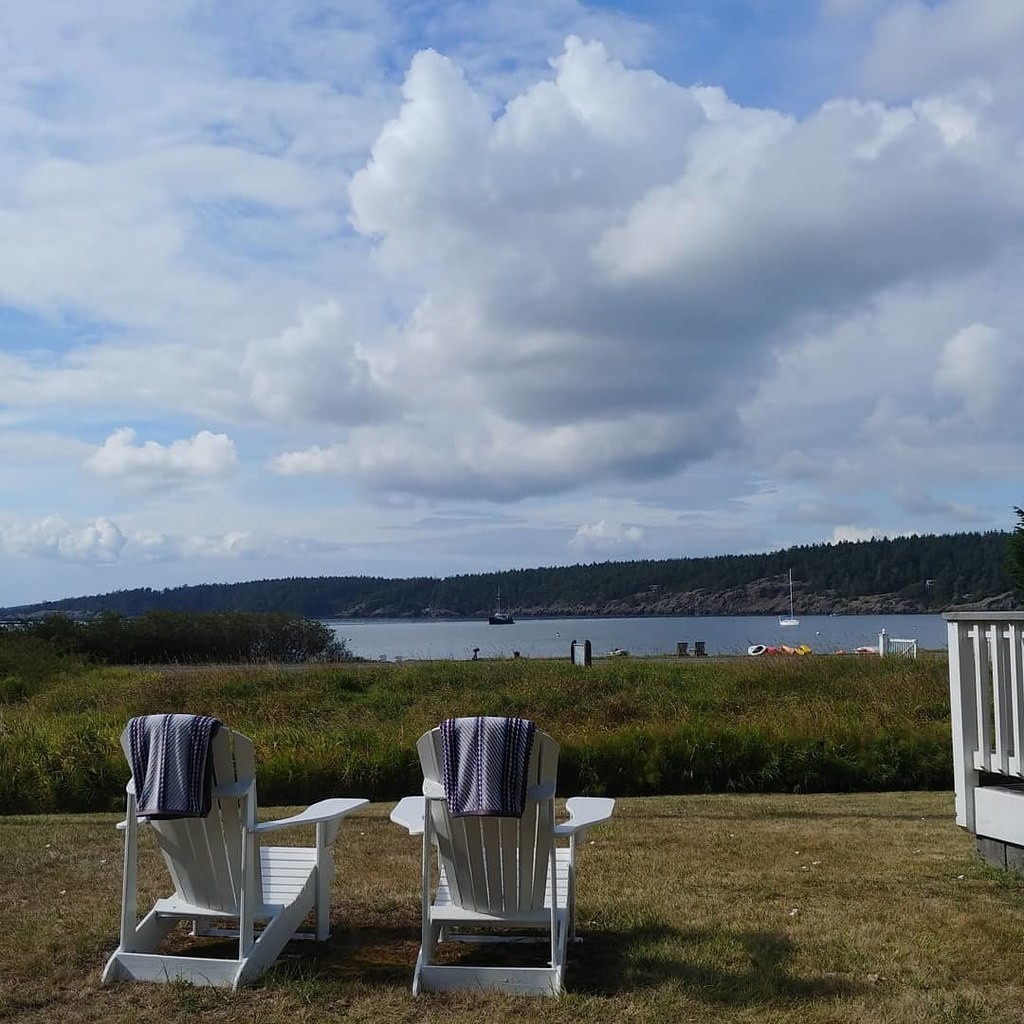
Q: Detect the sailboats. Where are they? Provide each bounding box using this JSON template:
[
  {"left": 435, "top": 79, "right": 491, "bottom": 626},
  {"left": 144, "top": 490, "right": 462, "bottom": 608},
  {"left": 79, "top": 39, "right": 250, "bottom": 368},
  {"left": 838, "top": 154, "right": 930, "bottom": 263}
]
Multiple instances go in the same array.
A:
[
  {"left": 777, "top": 568, "right": 801, "bottom": 627},
  {"left": 488, "top": 584, "right": 515, "bottom": 624}
]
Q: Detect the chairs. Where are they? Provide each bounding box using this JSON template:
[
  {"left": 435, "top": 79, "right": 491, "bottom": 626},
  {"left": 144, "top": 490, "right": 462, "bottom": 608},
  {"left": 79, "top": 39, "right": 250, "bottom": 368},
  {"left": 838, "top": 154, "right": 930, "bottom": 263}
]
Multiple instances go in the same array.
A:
[
  {"left": 673, "top": 642, "right": 689, "bottom": 658},
  {"left": 98, "top": 714, "right": 370, "bottom": 1000},
  {"left": 694, "top": 642, "right": 707, "bottom": 657},
  {"left": 388, "top": 716, "right": 614, "bottom": 997}
]
